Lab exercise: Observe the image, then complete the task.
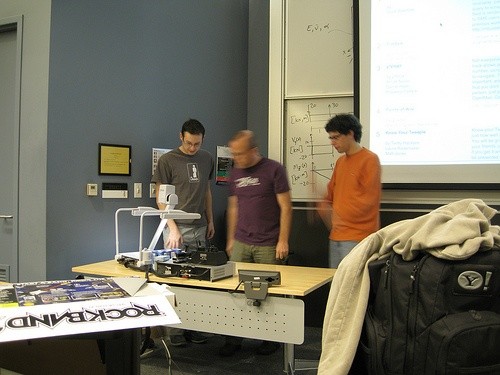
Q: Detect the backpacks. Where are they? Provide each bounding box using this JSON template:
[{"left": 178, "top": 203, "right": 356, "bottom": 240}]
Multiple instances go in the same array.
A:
[{"left": 353, "top": 241, "right": 500, "bottom": 375}]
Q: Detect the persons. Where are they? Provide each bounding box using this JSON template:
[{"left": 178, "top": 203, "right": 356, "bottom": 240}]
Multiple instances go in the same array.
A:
[
  {"left": 154, "top": 118, "right": 216, "bottom": 345},
  {"left": 219, "top": 129, "right": 293, "bottom": 356},
  {"left": 319, "top": 114, "right": 381, "bottom": 268}
]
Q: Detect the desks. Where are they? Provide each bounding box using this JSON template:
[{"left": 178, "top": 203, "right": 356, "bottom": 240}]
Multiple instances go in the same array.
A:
[
  {"left": 72, "top": 259, "right": 338, "bottom": 375},
  {"left": 0, "top": 276, "right": 176, "bottom": 375}
]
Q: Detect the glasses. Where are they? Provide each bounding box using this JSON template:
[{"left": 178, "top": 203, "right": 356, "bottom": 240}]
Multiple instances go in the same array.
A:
[{"left": 328, "top": 133, "right": 342, "bottom": 141}]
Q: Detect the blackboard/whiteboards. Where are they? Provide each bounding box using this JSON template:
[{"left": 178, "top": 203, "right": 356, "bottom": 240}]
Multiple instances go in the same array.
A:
[{"left": 279, "top": 0, "right": 500, "bottom": 207}]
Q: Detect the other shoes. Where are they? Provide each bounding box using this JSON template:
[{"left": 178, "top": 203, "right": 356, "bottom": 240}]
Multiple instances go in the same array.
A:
[
  {"left": 187, "top": 334, "right": 209, "bottom": 343},
  {"left": 169, "top": 335, "right": 187, "bottom": 346},
  {"left": 219, "top": 343, "right": 241, "bottom": 356},
  {"left": 255, "top": 342, "right": 279, "bottom": 354}
]
